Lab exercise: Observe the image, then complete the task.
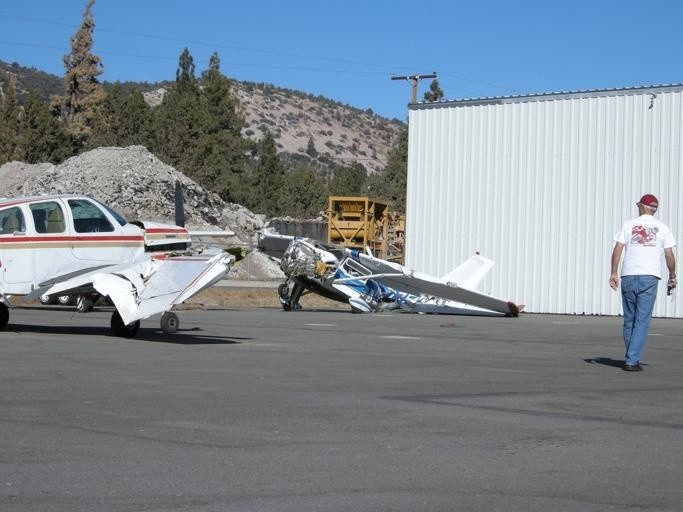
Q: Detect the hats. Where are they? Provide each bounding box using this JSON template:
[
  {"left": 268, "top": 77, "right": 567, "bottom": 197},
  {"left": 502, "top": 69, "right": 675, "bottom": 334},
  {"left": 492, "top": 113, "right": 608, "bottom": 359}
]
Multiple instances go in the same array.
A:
[{"left": 637, "top": 194, "right": 658, "bottom": 207}]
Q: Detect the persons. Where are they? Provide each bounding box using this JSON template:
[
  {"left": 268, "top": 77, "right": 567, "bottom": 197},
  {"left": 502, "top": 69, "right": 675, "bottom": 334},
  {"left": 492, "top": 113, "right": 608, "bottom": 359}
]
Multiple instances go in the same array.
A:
[{"left": 608, "top": 194, "right": 678, "bottom": 372}]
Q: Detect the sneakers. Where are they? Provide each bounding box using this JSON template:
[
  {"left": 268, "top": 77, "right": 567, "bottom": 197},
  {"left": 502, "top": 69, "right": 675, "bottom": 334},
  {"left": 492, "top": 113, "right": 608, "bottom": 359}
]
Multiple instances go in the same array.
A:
[{"left": 624, "top": 365, "right": 643, "bottom": 371}]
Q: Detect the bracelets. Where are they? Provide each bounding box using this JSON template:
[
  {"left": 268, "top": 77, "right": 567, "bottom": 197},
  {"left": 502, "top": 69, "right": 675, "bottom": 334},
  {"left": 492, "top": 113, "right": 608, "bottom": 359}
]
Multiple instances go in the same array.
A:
[{"left": 668, "top": 273, "right": 676, "bottom": 280}]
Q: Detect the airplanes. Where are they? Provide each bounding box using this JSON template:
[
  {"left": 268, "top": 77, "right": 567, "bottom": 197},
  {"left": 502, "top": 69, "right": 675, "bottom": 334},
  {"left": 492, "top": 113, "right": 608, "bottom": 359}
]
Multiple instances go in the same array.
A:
[
  {"left": 0, "top": 176, "right": 251, "bottom": 338},
  {"left": 257, "top": 219, "right": 526, "bottom": 317}
]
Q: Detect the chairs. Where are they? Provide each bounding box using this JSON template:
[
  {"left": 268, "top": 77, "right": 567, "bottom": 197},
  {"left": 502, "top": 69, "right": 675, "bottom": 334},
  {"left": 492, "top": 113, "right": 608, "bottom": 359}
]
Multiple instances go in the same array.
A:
[
  {"left": 31, "top": 209, "right": 64, "bottom": 233},
  {"left": 3, "top": 213, "right": 20, "bottom": 234}
]
